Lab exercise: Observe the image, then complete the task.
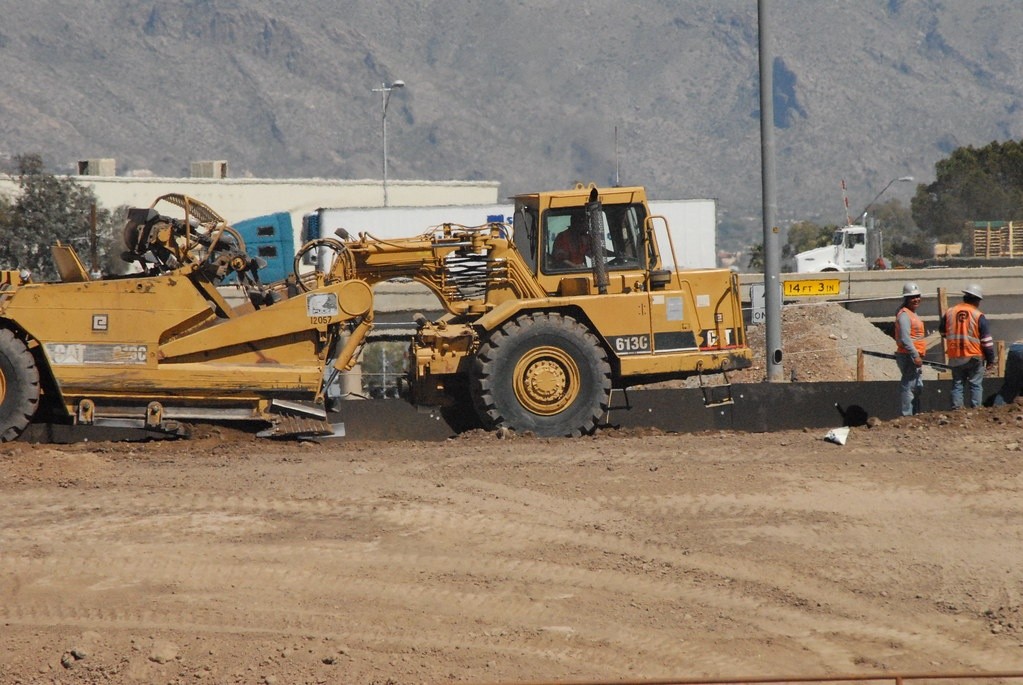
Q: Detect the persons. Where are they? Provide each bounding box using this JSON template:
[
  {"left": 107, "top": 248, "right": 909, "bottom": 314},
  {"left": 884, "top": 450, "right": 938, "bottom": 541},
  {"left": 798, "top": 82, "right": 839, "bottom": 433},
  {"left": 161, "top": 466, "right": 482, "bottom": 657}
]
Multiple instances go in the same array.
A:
[
  {"left": 894, "top": 282, "right": 926, "bottom": 416},
  {"left": 551, "top": 212, "right": 617, "bottom": 268},
  {"left": 940, "top": 286, "right": 994, "bottom": 410},
  {"left": 994, "top": 340, "right": 1023, "bottom": 404}
]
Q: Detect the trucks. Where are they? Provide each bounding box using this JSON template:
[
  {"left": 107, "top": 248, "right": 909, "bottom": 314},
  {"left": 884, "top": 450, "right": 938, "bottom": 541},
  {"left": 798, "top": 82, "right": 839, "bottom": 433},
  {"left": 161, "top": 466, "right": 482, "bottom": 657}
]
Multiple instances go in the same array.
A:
[
  {"left": 794, "top": 212, "right": 1023, "bottom": 273},
  {"left": 191, "top": 197, "right": 717, "bottom": 340}
]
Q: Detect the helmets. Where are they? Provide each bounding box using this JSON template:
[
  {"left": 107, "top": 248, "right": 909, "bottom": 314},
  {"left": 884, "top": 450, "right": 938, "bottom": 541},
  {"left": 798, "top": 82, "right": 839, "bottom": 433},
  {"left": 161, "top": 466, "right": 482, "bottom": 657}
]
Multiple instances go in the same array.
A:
[
  {"left": 962, "top": 284, "right": 984, "bottom": 299},
  {"left": 902, "top": 283, "right": 920, "bottom": 296}
]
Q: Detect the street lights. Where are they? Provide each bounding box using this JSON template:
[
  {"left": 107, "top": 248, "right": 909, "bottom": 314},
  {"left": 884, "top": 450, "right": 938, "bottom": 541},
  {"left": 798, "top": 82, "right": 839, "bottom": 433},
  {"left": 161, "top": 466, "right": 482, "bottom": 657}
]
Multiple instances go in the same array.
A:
[
  {"left": 371, "top": 80, "right": 406, "bottom": 208},
  {"left": 848, "top": 175, "right": 917, "bottom": 225}
]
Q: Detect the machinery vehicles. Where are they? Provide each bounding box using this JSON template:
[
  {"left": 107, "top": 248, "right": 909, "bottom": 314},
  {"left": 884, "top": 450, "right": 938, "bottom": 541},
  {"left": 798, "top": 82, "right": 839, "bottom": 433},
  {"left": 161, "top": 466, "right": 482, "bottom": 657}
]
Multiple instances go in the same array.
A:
[{"left": 0, "top": 181, "right": 755, "bottom": 446}]
